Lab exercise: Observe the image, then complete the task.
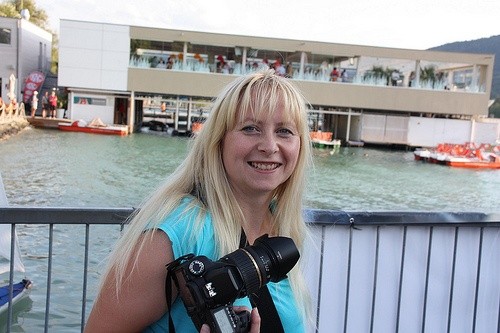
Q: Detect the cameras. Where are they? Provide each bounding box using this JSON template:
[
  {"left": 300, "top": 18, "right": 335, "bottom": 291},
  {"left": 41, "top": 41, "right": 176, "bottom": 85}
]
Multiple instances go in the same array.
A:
[{"left": 171, "top": 233, "right": 300, "bottom": 333}]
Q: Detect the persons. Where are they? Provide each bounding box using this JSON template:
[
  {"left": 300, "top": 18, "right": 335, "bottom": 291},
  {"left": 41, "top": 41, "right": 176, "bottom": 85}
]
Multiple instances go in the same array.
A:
[
  {"left": 31, "top": 89, "right": 57, "bottom": 118},
  {"left": 83, "top": 70, "right": 320, "bottom": 333},
  {"left": 147, "top": 54, "right": 452, "bottom": 91}
]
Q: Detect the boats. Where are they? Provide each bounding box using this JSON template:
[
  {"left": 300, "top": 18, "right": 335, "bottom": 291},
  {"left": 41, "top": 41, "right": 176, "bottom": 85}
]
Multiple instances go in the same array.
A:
[
  {"left": 413, "top": 143, "right": 500, "bottom": 168},
  {"left": 59, "top": 118, "right": 129, "bottom": 134},
  {"left": 311, "top": 140, "right": 342, "bottom": 150}
]
